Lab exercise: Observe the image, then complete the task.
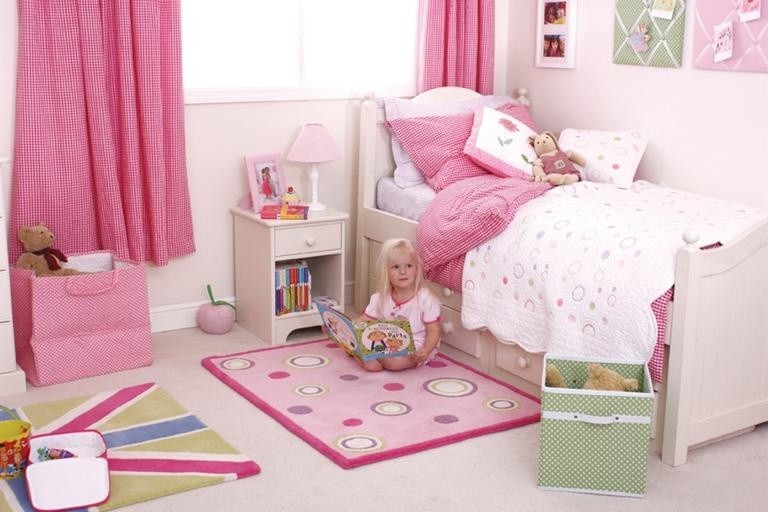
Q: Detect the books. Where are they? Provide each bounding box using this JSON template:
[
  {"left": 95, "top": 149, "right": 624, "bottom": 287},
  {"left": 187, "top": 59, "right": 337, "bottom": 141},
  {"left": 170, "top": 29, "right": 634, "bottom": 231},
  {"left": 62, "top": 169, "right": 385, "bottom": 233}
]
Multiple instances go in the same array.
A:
[
  {"left": 317, "top": 303, "right": 416, "bottom": 363},
  {"left": 276, "top": 261, "right": 311, "bottom": 317},
  {"left": 260, "top": 204, "right": 310, "bottom": 220}
]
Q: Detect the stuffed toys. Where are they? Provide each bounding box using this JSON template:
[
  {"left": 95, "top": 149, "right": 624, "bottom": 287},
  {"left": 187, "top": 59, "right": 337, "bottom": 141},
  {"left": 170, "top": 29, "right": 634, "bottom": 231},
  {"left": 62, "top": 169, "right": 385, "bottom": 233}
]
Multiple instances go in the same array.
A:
[
  {"left": 530, "top": 132, "right": 587, "bottom": 187},
  {"left": 545, "top": 362, "right": 639, "bottom": 394},
  {"left": 15, "top": 221, "right": 85, "bottom": 277}
]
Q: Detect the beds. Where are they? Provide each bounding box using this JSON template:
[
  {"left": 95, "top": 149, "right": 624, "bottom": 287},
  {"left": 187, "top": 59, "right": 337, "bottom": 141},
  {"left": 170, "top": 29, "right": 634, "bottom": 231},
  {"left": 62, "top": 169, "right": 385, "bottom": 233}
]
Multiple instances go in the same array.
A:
[{"left": 354, "top": 86, "right": 768, "bottom": 466}]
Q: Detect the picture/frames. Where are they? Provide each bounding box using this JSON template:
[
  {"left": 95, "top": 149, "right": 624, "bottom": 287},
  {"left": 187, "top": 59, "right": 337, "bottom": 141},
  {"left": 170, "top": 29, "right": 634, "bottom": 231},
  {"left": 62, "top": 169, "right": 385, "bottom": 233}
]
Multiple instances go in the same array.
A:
[
  {"left": 244, "top": 153, "right": 289, "bottom": 213},
  {"left": 535, "top": 0, "right": 577, "bottom": 68}
]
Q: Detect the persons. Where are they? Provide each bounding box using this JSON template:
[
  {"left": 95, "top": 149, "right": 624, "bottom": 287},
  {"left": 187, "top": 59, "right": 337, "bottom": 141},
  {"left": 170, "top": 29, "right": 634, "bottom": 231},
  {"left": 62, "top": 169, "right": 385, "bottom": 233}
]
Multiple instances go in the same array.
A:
[
  {"left": 544, "top": 34, "right": 565, "bottom": 58},
  {"left": 261, "top": 166, "right": 279, "bottom": 199},
  {"left": 320, "top": 238, "right": 443, "bottom": 373}
]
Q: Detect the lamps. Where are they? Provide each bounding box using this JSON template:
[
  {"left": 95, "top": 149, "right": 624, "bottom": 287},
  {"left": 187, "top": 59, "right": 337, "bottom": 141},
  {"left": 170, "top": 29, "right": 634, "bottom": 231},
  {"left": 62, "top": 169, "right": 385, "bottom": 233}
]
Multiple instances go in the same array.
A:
[{"left": 287, "top": 124, "right": 341, "bottom": 212}]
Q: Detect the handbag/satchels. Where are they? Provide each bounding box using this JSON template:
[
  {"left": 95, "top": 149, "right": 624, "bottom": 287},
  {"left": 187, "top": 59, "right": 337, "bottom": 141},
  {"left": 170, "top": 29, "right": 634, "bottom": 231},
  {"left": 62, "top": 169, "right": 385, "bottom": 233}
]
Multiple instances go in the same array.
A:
[{"left": 9, "top": 248, "right": 153, "bottom": 388}]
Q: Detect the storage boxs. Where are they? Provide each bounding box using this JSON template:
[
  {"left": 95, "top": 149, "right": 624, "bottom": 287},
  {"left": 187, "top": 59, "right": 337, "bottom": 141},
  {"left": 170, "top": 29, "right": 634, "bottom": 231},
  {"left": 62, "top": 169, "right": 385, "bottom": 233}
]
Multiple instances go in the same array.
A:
[{"left": 538, "top": 353, "right": 654, "bottom": 497}]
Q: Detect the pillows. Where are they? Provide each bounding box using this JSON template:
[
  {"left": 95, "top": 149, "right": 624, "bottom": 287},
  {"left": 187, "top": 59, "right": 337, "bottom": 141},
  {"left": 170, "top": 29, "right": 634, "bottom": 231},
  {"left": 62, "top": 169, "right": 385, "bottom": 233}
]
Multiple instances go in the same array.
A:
[
  {"left": 382, "top": 94, "right": 521, "bottom": 188},
  {"left": 555, "top": 126, "right": 648, "bottom": 189},
  {"left": 384, "top": 104, "right": 540, "bottom": 189},
  {"left": 461, "top": 104, "right": 538, "bottom": 181}
]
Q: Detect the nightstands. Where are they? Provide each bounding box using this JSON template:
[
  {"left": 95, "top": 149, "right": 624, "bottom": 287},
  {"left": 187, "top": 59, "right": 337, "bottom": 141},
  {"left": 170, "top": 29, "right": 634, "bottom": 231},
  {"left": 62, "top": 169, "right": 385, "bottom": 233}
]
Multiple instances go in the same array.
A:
[{"left": 230, "top": 207, "right": 351, "bottom": 346}]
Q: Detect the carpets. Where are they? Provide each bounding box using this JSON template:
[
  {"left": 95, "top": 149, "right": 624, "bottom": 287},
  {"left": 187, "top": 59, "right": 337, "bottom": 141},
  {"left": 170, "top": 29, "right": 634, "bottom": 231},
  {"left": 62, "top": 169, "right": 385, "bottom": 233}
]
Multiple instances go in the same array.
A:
[
  {"left": 201, "top": 339, "right": 541, "bottom": 470},
  {"left": 0, "top": 383, "right": 261, "bottom": 512}
]
